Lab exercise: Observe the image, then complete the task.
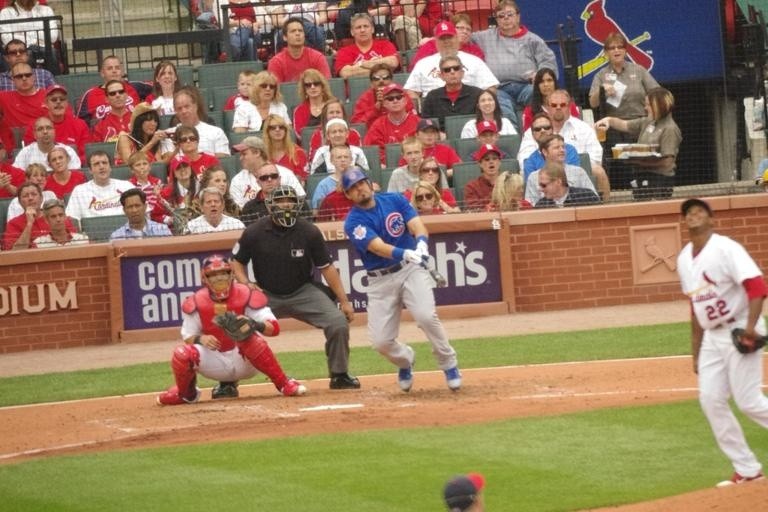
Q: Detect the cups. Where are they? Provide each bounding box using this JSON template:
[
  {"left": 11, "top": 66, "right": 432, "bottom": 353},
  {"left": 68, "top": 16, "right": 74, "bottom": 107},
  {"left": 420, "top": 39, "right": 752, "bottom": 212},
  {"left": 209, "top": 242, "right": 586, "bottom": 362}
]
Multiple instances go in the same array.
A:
[
  {"left": 597, "top": 126, "right": 607, "bottom": 142},
  {"left": 612, "top": 143, "right": 660, "bottom": 158}
]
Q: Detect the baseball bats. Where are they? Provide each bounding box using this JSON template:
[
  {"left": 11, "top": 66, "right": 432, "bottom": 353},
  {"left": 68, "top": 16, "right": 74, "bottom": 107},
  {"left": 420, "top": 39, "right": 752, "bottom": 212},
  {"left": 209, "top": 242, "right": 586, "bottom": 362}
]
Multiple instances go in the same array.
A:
[{"left": 422, "top": 260, "right": 446, "bottom": 289}]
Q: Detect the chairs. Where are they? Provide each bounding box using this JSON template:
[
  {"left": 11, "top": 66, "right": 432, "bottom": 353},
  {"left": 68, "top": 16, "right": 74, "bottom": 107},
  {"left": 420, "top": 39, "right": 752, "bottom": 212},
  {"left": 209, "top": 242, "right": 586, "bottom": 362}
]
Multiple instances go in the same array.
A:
[
  {"left": 55, "top": 59, "right": 608, "bottom": 243},
  {"left": 454, "top": 1, "right": 498, "bottom": 33}
]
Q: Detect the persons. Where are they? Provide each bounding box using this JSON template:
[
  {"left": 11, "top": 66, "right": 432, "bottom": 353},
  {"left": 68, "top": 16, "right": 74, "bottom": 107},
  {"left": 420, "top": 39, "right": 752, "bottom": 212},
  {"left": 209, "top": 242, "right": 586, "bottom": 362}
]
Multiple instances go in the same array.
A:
[
  {"left": 673, "top": 195, "right": 768, "bottom": 492},
  {"left": 155, "top": 251, "right": 309, "bottom": 404},
  {"left": 211, "top": 184, "right": 362, "bottom": 398},
  {"left": 341, "top": 164, "right": 463, "bottom": 394}
]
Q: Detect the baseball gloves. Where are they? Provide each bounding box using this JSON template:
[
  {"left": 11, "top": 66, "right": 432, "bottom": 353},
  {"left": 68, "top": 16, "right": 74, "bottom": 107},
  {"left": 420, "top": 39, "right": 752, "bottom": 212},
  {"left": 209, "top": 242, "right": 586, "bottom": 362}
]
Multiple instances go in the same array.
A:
[
  {"left": 212, "top": 312, "right": 255, "bottom": 341},
  {"left": 731, "top": 328, "right": 768, "bottom": 353}
]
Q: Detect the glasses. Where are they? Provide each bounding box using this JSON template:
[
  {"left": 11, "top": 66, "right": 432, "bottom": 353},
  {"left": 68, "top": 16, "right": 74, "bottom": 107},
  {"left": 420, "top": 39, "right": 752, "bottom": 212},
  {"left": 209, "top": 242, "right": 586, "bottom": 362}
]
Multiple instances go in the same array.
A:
[{"left": 0, "top": 11, "right": 627, "bottom": 206}]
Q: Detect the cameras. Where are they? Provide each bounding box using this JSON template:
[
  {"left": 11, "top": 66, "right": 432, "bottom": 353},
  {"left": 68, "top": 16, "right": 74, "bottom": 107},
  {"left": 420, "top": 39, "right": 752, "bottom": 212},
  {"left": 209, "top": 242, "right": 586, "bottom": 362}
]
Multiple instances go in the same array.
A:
[{"left": 165, "top": 132, "right": 176, "bottom": 138}]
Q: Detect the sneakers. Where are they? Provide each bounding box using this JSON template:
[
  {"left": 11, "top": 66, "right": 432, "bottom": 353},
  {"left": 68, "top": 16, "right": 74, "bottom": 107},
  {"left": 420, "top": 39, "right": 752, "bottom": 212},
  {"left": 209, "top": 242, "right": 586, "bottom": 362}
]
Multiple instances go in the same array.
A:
[
  {"left": 328, "top": 372, "right": 361, "bottom": 390},
  {"left": 715, "top": 472, "right": 765, "bottom": 488},
  {"left": 397, "top": 360, "right": 417, "bottom": 392},
  {"left": 156, "top": 386, "right": 202, "bottom": 406},
  {"left": 212, "top": 381, "right": 239, "bottom": 399},
  {"left": 280, "top": 378, "right": 307, "bottom": 397},
  {"left": 445, "top": 366, "right": 463, "bottom": 391}
]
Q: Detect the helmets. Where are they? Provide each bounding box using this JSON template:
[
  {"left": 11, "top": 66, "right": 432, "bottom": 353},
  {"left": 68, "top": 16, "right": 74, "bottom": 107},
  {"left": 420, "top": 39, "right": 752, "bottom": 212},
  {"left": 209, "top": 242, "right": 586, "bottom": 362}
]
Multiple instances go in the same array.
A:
[
  {"left": 199, "top": 254, "right": 236, "bottom": 301},
  {"left": 264, "top": 185, "right": 306, "bottom": 229}
]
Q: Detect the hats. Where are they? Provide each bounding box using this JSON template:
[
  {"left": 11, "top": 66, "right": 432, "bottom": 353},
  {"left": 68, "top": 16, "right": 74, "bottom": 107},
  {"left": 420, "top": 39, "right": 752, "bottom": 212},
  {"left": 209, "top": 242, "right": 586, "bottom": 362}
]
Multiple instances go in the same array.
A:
[{"left": 681, "top": 198, "right": 713, "bottom": 219}]
